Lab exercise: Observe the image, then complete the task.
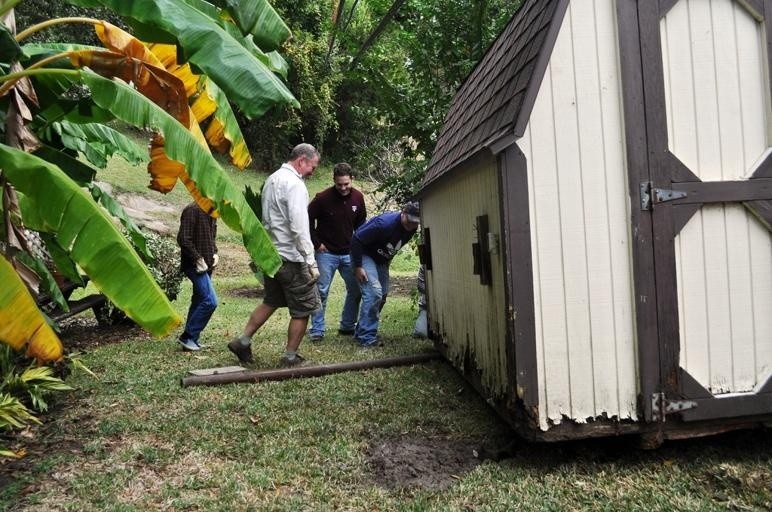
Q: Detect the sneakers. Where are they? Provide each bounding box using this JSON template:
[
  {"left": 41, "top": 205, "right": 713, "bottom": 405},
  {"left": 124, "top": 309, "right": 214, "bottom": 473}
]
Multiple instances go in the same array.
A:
[
  {"left": 372, "top": 341, "right": 383, "bottom": 346},
  {"left": 178, "top": 334, "right": 201, "bottom": 351},
  {"left": 308, "top": 329, "right": 324, "bottom": 341},
  {"left": 282, "top": 353, "right": 305, "bottom": 365},
  {"left": 339, "top": 326, "right": 356, "bottom": 334},
  {"left": 228, "top": 338, "right": 255, "bottom": 363}
]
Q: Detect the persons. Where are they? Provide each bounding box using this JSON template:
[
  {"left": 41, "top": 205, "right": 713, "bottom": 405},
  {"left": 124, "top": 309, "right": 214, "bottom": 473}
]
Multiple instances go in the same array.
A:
[
  {"left": 176, "top": 201, "right": 220, "bottom": 351},
  {"left": 308, "top": 163, "right": 366, "bottom": 341},
  {"left": 227, "top": 142, "right": 324, "bottom": 367},
  {"left": 350, "top": 201, "right": 420, "bottom": 347}
]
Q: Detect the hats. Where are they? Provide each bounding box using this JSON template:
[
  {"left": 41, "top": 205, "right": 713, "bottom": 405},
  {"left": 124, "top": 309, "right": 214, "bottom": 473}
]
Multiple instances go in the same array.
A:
[{"left": 405, "top": 202, "right": 420, "bottom": 223}]
